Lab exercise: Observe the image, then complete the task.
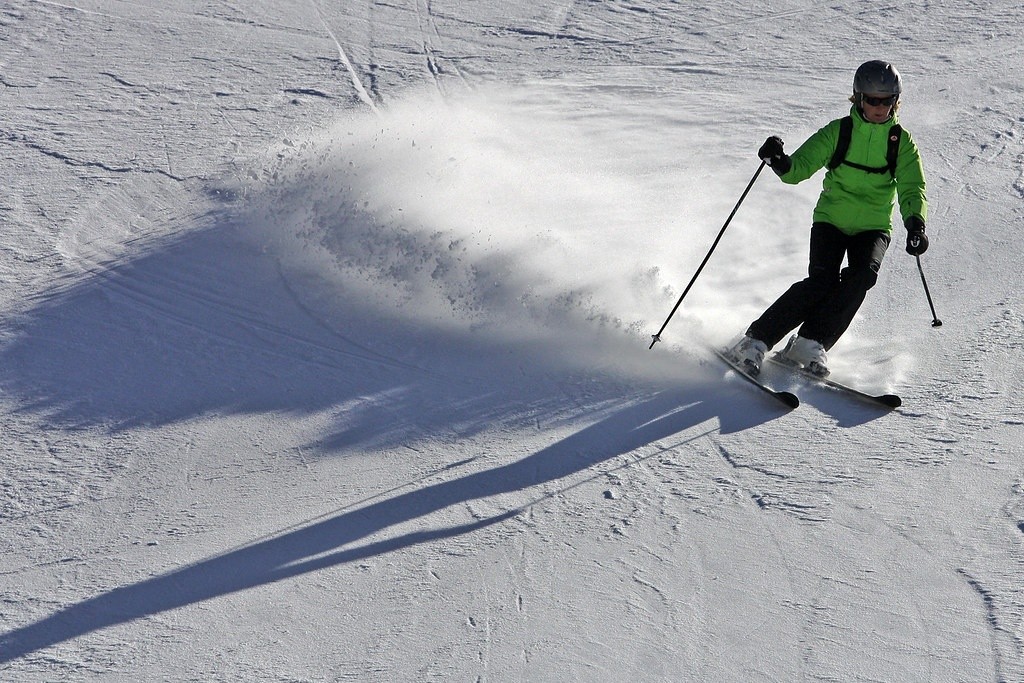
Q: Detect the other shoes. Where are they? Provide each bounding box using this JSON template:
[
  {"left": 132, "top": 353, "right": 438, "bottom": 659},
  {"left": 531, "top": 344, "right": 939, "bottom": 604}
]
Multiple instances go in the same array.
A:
[
  {"left": 731, "top": 338, "right": 767, "bottom": 377},
  {"left": 785, "top": 335, "right": 831, "bottom": 377}
]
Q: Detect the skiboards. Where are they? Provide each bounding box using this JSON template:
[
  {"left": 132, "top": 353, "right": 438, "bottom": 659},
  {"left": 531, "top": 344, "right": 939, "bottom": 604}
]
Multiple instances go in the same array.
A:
[{"left": 707, "top": 341, "right": 904, "bottom": 419}]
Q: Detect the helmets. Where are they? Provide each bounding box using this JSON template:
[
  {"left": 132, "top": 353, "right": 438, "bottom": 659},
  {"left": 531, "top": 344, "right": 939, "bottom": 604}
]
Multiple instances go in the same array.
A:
[{"left": 853, "top": 60, "right": 903, "bottom": 109}]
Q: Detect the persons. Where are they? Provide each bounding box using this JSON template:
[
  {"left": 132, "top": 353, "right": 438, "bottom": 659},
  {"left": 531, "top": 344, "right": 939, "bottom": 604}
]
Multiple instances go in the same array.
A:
[{"left": 718, "top": 60, "right": 930, "bottom": 380}]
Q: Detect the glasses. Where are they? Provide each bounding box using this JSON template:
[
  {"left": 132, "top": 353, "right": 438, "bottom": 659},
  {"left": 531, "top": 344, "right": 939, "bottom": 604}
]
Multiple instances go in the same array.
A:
[{"left": 863, "top": 95, "right": 897, "bottom": 106}]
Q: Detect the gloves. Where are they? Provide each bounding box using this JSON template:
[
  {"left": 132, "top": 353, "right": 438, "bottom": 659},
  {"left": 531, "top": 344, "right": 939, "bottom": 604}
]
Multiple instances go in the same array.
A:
[
  {"left": 904, "top": 215, "right": 929, "bottom": 256},
  {"left": 758, "top": 136, "right": 793, "bottom": 176}
]
